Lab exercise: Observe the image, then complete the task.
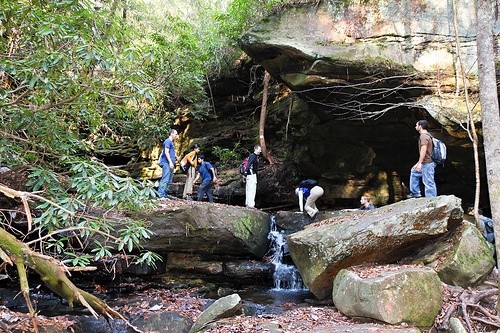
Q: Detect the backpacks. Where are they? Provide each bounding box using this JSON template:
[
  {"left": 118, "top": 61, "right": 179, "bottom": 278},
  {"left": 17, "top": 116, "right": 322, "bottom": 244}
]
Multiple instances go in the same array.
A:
[
  {"left": 300, "top": 179, "right": 318, "bottom": 189},
  {"left": 479, "top": 217, "right": 495, "bottom": 245},
  {"left": 180, "top": 152, "right": 197, "bottom": 173},
  {"left": 420, "top": 133, "right": 447, "bottom": 164},
  {"left": 210, "top": 166, "right": 217, "bottom": 178},
  {"left": 239, "top": 154, "right": 257, "bottom": 175}
]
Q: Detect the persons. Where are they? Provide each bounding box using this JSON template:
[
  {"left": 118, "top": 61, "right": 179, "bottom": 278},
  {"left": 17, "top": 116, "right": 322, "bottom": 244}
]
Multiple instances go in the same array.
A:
[
  {"left": 157, "top": 129, "right": 177, "bottom": 200},
  {"left": 407, "top": 121, "right": 437, "bottom": 197},
  {"left": 245, "top": 145, "right": 261, "bottom": 209},
  {"left": 193, "top": 155, "right": 217, "bottom": 203},
  {"left": 468, "top": 206, "right": 488, "bottom": 240},
  {"left": 340, "top": 196, "right": 372, "bottom": 212},
  {"left": 183, "top": 143, "right": 200, "bottom": 200},
  {"left": 296, "top": 186, "right": 324, "bottom": 221}
]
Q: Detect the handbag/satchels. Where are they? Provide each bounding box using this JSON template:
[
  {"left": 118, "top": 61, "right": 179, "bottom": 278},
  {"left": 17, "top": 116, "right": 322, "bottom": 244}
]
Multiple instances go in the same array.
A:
[{"left": 156, "top": 150, "right": 163, "bottom": 168}]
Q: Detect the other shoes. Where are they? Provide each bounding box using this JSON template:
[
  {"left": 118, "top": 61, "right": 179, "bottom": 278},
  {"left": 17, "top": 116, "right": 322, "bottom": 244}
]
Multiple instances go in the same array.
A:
[
  {"left": 407, "top": 195, "right": 422, "bottom": 198},
  {"left": 311, "top": 210, "right": 320, "bottom": 221},
  {"left": 246, "top": 206, "right": 257, "bottom": 210}
]
